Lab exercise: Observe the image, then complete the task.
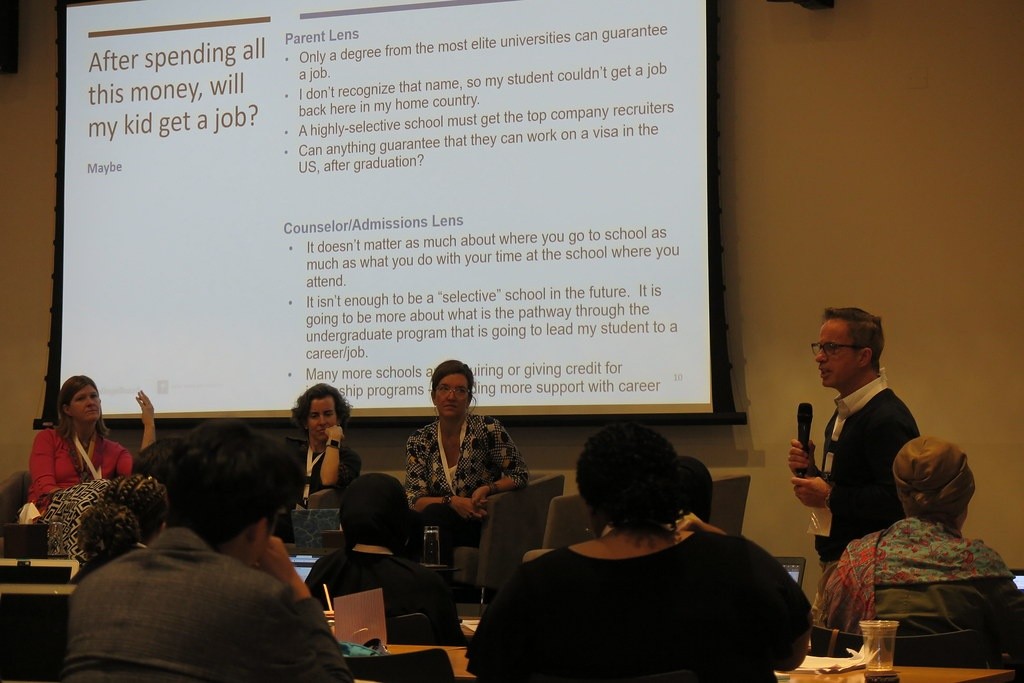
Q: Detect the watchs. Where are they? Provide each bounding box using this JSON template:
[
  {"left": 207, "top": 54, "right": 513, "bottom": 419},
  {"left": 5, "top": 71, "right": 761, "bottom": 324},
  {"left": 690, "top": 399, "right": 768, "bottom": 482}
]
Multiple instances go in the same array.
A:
[
  {"left": 326, "top": 439, "right": 340, "bottom": 448},
  {"left": 442, "top": 494, "right": 452, "bottom": 505},
  {"left": 825, "top": 493, "right": 830, "bottom": 510}
]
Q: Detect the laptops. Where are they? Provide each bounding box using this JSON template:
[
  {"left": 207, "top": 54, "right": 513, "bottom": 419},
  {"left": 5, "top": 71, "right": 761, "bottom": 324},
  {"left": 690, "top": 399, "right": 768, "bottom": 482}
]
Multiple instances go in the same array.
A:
[
  {"left": 288, "top": 553, "right": 323, "bottom": 583},
  {"left": 773, "top": 557, "right": 806, "bottom": 589}
]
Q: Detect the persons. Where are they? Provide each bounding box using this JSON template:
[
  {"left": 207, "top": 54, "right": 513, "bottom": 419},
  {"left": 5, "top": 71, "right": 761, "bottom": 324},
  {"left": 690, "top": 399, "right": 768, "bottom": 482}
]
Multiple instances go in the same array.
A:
[
  {"left": 788, "top": 306, "right": 922, "bottom": 571},
  {"left": 305, "top": 472, "right": 467, "bottom": 646},
  {"left": 41, "top": 438, "right": 176, "bottom": 565},
  {"left": 28, "top": 375, "right": 157, "bottom": 523},
  {"left": 463, "top": 421, "right": 813, "bottom": 683},
  {"left": 269, "top": 384, "right": 362, "bottom": 544},
  {"left": 676, "top": 455, "right": 713, "bottom": 522},
  {"left": 76, "top": 502, "right": 143, "bottom": 559},
  {"left": 817, "top": 438, "right": 1024, "bottom": 664},
  {"left": 405, "top": 360, "right": 530, "bottom": 546},
  {"left": 100, "top": 473, "right": 169, "bottom": 544},
  {"left": 65, "top": 423, "right": 353, "bottom": 683}
]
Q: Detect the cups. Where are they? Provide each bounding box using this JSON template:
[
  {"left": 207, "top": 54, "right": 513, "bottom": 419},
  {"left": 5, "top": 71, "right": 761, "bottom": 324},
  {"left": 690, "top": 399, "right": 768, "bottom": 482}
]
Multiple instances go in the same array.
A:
[
  {"left": 859, "top": 620, "right": 900, "bottom": 672},
  {"left": 48, "top": 519, "right": 64, "bottom": 556},
  {"left": 422, "top": 525, "right": 441, "bottom": 565}
]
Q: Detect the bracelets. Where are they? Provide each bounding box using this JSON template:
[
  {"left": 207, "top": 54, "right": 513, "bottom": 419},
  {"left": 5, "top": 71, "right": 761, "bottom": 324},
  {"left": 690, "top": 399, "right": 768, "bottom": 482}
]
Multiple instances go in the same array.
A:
[{"left": 482, "top": 482, "right": 498, "bottom": 495}]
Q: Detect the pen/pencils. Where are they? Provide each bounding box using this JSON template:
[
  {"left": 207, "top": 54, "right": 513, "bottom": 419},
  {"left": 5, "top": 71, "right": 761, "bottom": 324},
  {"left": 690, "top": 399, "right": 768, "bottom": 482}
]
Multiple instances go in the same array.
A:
[
  {"left": 480, "top": 587, "right": 485, "bottom": 604},
  {"left": 137, "top": 542, "right": 148, "bottom": 548},
  {"left": 323, "top": 584, "right": 333, "bottom": 611}
]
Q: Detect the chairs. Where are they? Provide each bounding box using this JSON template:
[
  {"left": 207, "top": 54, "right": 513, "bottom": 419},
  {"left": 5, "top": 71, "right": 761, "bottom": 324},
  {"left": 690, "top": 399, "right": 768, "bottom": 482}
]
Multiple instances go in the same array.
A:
[{"left": 0, "top": 472, "right": 752, "bottom": 683}]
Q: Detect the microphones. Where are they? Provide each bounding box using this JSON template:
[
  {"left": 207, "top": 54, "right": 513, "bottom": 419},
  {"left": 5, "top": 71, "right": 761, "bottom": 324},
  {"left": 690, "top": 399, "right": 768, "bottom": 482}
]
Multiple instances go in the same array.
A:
[{"left": 796, "top": 403, "right": 813, "bottom": 478}]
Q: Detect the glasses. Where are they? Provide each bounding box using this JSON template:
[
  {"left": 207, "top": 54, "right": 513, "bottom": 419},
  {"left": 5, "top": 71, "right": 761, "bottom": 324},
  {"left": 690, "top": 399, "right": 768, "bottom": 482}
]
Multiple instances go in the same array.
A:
[
  {"left": 811, "top": 342, "right": 862, "bottom": 356},
  {"left": 434, "top": 384, "right": 471, "bottom": 400}
]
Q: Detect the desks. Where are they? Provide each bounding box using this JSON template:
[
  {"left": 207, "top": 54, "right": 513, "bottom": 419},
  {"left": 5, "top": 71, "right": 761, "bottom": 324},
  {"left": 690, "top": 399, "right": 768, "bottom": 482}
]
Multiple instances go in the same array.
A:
[
  {"left": 349, "top": 642, "right": 476, "bottom": 683},
  {"left": 772, "top": 662, "right": 1017, "bottom": 683}
]
